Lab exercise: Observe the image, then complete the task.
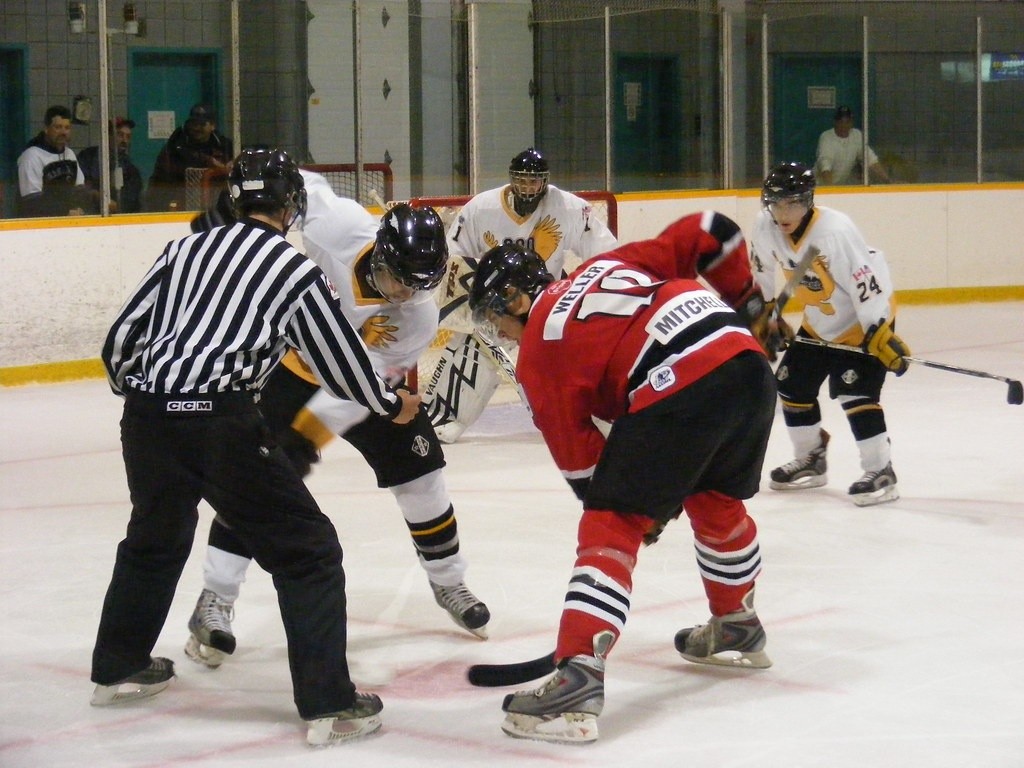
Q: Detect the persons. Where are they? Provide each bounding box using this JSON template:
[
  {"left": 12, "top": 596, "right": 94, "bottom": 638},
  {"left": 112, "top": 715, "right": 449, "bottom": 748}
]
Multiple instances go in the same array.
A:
[
  {"left": 17, "top": 104, "right": 234, "bottom": 217},
  {"left": 420, "top": 147, "right": 617, "bottom": 444},
  {"left": 750, "top": 158, "right": 912, "bottom": 494},
  {"left": 186, "top": 164, "right": 491, "bottom": 653},
  {"left": 91, "top": 148, "right": 421, "bottom": 721},
  {"left": 468, "top": 210, "right": 798, "bottom": 717},
  {"left": 812, "top": 104, "right": 893, "bottom": 186}
]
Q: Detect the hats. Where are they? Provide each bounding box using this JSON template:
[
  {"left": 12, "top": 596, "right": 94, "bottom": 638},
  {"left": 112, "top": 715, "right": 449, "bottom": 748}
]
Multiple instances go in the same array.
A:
[
  {"left": 108, "top": 117, "right": 136, "bottom": 133},
  {"left": 185, "top": 104, "right": 215, "bottom": 124},
  {"left": 834, "top": 105, "right": 851, "bottom": 119}
]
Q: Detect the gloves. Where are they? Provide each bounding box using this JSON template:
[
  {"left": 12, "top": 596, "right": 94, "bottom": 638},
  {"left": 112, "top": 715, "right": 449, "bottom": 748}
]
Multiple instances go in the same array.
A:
[
  {"left": 862, "top": 317, "right": 911, "bottom": 377},
  {"left": 750, "top": 298, "right": 788, "bottom": 362},
  {"left": 773, "top": 316, "right": 795, "bottom": 342}
]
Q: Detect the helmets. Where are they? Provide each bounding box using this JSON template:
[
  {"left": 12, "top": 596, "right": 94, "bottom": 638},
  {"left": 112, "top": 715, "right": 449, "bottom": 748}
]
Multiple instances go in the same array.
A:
[
  {"left": 468, "top": 243, "right": 555, "bottom": 327},
  {"left": 508, "top": 147, "right": 550, "bottom": 179},
  {"left": 223, "top": 147, "right": 305, "bottom": 218},
  {"left": 761, "top": 160, "right": 816, "bottom": 211},
  {"left": 377, "top": 197, "right": 449, "bottom": 291}
]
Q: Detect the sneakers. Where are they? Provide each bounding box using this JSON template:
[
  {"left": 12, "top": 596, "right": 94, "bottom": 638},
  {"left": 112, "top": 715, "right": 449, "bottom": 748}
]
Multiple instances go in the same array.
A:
[
  {"left": 674, "top": 581, "right": 772, "bottom": 669},
  {"left": 501, "top": 630, "right": 615, "bottom": 745},
  {"left": 769, "top": 428, "right": 830, "bottom": 490},
  {"left": 429, "top": 579, "right": 490, "bottom": 640},
  {"left": 90, "top": 657, "right": 175, "bottom": 705},
  {"left": 848, "top": 461, "right": 899, "bottom": 507},
  {"left": 185, "top": 589, "right": 236, "bottom": 669},
  {"left": 300, "top": 692, "right": 383, "bottom": 746}
]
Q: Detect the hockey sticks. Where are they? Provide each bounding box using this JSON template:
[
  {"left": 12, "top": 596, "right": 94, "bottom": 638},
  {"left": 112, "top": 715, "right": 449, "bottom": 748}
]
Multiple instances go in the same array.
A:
[
  {"left": 467, "top": 242, "right": 822, "bottom": 688},
  {"left": 795, "top": 335, "right": 1024, "bottom": 405},
  {"left": 368, "top": 186, "right": 534, "bottom": 419}
]
